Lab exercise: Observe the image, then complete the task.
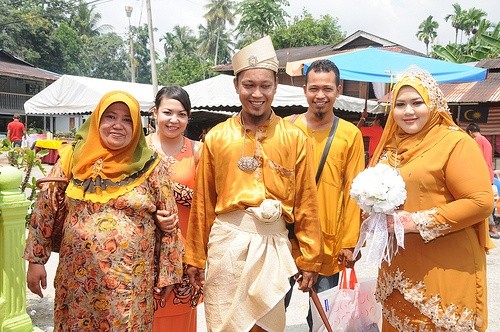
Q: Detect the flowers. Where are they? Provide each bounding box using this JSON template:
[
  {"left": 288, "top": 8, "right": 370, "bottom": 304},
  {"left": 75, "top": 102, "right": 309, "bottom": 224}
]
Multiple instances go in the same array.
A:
[{"left": 349, "top": 163, "right": 408, "bottom": 271}]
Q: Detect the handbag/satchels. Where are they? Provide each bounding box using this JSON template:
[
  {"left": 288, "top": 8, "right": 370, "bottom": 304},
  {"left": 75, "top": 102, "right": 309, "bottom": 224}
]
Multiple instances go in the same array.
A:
[
  {"left": 310, "top": 272, "right": 342, "bottom": 332},
  {"left": 319, "top": 265, "right": 382, "bottom": 332},
  {"left": 36, "top": 178, "right": 69, "bottom": 252}
]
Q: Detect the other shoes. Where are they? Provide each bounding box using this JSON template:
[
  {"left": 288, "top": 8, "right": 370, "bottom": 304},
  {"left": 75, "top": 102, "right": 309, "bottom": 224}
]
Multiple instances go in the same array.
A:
[{"left": 491, "top": 234, "right": 499, "bottom": 239}]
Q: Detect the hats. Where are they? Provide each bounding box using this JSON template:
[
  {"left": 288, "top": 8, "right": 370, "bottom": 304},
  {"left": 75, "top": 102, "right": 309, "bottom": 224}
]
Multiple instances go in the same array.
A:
[{"left": 232, "top": 35, "right": 279, "bottom": 75}]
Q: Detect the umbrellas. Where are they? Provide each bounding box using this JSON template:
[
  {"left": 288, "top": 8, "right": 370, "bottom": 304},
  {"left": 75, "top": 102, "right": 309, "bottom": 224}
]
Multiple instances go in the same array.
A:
[{"left": 285, "top": 47, "right": 488, "bottom": 97}]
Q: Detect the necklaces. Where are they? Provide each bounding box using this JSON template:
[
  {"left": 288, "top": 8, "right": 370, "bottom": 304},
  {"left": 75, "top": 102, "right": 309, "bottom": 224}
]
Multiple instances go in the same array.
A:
[
  {"left": 147, "top": 133, "right": 188, "bottom": 170},
  {"left": 305, "top": 116, "right": 331, "bottom": 133},
  {"left": 237, "top": 112, "right": 262, "bottom": 172}
]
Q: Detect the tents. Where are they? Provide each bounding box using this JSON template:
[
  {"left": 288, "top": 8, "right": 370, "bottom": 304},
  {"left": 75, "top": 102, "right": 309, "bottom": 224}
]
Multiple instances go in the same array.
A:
[{"left": 25, "top": 73, "right": 391, "bottom": 169}]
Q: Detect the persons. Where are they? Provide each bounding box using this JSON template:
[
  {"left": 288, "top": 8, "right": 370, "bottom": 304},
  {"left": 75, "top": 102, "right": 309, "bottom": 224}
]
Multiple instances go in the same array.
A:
[
  {"left": 464, "top": 122, "right": 500, "bottom": 239},
  {"left": 22, "top": 90, "right": 184, "bottom": 331},
  {"left": 280, "top": 59, "right": 366, "bottom": 332},
  {"left": 366, "top": 69, "right": 495, "bottom": 332},
  {"left": 357, "top": 104, "right": 386, "bottom": 167},
  {"left": 144, "top": 86, "right": 205, "bottom": 332},
  {"left": 5, "top": 114, "right": 26, "bottom": 167},
  {"left": 184, "top": 34, "right": 322, "bottom": 332}
]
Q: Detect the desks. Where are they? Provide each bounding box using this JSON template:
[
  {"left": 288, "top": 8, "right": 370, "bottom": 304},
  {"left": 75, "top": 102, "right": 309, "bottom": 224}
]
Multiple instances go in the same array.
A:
[{"left": 22, "top": 134, "right": 75, "bottom": 165}]
